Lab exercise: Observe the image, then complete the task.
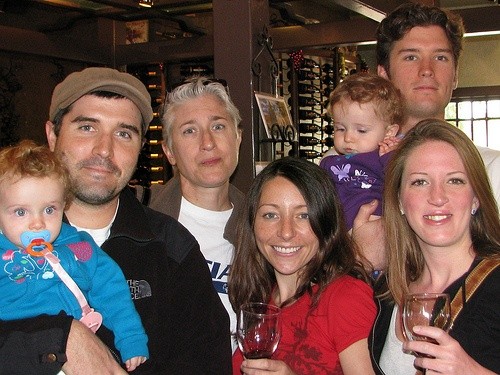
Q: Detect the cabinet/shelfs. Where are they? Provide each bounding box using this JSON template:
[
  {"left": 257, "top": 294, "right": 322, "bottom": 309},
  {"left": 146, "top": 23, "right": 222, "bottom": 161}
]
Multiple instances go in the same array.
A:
[
  {"left": 126, "top": 57, "right": 180, "bottom": 187},
  {"left": 290, "top": 49, "right": 342, "bottom": 166}
]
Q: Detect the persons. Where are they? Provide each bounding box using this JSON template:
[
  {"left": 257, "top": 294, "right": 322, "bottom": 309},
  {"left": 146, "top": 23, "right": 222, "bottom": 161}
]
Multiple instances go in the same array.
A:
[
  {"left": 0, "top": 140, "right": 149, "bottom": 375},
  {"left": 320, "top": 0, "right": 500, "bottom": 288},
  {"left": 368, "top": 120, "right": 499, "bottom": 375},
  {"left": 228, "top": 156, "right": 380, "bottom": 375},
  {"left": 319, "top": 73, "right": 405, "bottom": 237},
  {"left": 149, "top": 80, "right": 261, "bottom": 354},
  {"left": 0, "top": 66, "right": 232, "bottom": 375}
]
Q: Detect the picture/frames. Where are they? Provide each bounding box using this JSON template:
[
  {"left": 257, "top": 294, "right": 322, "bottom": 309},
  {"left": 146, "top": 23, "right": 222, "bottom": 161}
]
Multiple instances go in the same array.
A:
[{"left": 255, "top": 90, "right": 297, "bottom": 141}]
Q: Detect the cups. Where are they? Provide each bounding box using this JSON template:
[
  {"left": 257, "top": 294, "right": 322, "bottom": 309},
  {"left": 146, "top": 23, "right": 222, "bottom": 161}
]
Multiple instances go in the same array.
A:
[
  {"left": 401, "top": 293, "right": 452, "bottom": 359},
  {"left": 236, "top": 302, "right": 282, "bottom": 358}
]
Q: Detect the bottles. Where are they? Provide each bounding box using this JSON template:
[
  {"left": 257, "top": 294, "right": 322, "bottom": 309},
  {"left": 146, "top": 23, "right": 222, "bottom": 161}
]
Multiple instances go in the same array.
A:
[
  {"left": 154, "top": 31, "right": 192, "bottom": 39},
  {"left": 126, "top": 65, "right": 220, "bottom": 187},
  {"left": 286, "top": 57, "right": 349, "bottom": 160}
]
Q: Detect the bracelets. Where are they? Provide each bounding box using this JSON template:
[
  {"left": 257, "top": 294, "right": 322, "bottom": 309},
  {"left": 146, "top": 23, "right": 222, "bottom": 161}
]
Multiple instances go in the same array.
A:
[{"left": 371, "top": 270, "right": 381, "bottom": 285}]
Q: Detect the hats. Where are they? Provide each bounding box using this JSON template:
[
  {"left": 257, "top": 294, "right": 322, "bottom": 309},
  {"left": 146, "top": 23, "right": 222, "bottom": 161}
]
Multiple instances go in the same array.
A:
[{"left": 48, "top": 68, "right": 154, "bottom": 134}]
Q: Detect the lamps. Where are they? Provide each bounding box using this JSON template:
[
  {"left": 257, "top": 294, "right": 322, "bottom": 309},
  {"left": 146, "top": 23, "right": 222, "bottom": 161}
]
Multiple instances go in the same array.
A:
[{"left": 138, "top": 0, "right": 153, "bottom": 8}]
